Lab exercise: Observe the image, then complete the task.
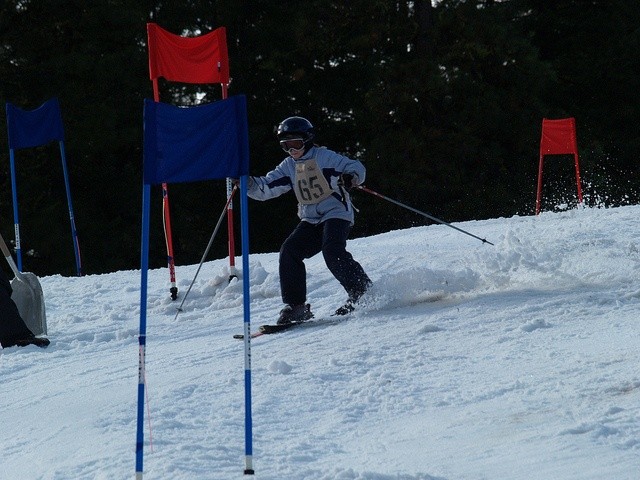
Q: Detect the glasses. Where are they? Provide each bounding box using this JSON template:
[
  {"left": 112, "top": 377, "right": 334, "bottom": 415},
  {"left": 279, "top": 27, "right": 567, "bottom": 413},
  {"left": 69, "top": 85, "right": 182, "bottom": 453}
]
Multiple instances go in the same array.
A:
[{"left": 279, "top": 137, "right": 314, "bottom": 152}]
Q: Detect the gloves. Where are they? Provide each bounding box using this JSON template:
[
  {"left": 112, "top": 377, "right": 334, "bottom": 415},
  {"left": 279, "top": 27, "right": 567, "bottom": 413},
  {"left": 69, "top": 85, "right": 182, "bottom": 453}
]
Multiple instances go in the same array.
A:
[{"left": 343, "top": 173, "right": 358, "bottom": 188}]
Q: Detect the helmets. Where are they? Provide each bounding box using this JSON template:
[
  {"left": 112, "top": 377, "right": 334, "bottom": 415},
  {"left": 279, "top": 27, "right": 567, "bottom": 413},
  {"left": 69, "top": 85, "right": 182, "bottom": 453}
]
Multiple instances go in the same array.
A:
[{"left": 277, "top": 116, "right": 314, "bottom": 142}]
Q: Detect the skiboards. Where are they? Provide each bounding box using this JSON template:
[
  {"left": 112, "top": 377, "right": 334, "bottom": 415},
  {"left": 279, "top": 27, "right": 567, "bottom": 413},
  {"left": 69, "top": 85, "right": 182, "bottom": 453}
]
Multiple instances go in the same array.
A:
[{"left": 231, "top": 295, "right": 363, "bottom": 339}]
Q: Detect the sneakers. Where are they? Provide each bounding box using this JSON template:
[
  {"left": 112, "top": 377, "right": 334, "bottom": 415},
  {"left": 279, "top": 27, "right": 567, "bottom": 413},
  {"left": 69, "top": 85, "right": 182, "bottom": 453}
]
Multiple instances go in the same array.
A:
[
  {"left": 277, "top": 304, "right": 306, "bottom": 325},
  {"left": 346, "top": 292, "right": 364, "bottom": 304}
]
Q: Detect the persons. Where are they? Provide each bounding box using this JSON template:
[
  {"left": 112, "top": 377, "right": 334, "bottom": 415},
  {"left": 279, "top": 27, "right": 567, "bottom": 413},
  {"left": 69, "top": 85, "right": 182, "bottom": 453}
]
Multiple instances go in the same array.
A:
[{"left": 235, "top": 116, "right": 372, "bottom": 325}]
